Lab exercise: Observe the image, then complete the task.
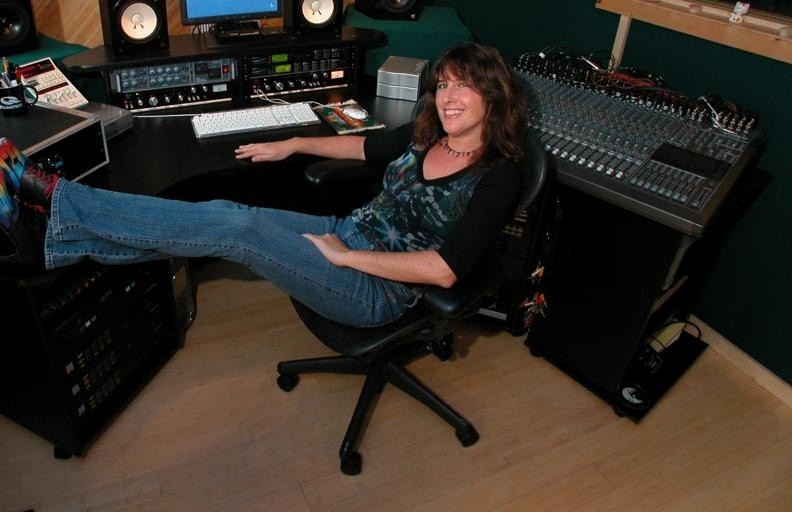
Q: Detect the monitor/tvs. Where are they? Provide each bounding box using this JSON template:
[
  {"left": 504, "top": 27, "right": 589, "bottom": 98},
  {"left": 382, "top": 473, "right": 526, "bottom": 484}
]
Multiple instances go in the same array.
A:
[{"left": 178, "top": 0, "right": 281, "bottom": 48}]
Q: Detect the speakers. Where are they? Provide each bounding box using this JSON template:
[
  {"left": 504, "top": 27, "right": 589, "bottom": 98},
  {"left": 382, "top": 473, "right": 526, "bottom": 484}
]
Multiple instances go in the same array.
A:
[
  {"left": 98, "top": 0, "right": 170, "bottom": 58},
  {"left": 281, "top": 0, "right": 344, "bottom": 42}
]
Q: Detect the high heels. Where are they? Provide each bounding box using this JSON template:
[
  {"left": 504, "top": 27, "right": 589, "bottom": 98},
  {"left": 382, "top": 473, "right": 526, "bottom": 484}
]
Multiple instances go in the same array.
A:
[
  {"left": 0, "top": 168, "right": 51, "bottom": 267},
  {"left": 0, "top": 137, "right": 59, "bottom": 216}
]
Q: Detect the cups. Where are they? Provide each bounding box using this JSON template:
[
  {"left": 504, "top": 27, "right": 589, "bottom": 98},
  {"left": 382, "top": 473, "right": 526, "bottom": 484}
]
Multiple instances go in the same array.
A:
[{"left": 0, "top": 82, "right": 38, "bottom": 117}]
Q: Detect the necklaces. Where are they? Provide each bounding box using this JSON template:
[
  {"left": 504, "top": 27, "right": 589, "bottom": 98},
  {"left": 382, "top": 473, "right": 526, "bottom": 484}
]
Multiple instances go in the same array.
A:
[{"left": 441, "top": 136, "right": 484, "bottom": 159}]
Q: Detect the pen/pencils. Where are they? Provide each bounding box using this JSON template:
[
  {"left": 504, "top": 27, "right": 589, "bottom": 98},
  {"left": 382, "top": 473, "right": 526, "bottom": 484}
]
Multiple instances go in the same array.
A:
[{"left": 0, "top": 56, "right": 22, "bottom": 87}]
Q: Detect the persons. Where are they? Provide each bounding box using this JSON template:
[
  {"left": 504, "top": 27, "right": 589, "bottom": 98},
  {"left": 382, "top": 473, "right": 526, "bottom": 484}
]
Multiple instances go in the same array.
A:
[{"left": 0, "top": 43, "right": 527, "bottom": 331}]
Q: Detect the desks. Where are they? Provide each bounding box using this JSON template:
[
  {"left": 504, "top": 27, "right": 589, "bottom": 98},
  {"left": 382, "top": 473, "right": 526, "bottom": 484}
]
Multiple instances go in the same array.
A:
[{"left": 84, "top": 76, "right": 420, "bottom": 272}]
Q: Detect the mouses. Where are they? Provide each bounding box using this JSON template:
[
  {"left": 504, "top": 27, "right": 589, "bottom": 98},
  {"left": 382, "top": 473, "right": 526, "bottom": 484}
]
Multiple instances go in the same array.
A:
[{"left": 342, "top": 106, "right": 368, "bottom": 120}]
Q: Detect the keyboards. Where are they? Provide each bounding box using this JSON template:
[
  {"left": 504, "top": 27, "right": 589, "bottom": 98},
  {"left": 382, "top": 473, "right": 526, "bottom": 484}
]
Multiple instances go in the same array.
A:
[{"left": 192, "top": 102, "right": 322, "bottom": 138}]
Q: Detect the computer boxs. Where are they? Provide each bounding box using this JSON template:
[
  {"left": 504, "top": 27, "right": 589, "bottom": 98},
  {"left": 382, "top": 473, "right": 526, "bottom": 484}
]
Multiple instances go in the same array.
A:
[{"left": 153, "top": 253, "right": 196, "bottom": 333}]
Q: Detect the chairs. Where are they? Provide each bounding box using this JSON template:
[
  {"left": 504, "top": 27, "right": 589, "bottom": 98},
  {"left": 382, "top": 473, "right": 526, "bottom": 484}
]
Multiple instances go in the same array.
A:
[{"left": 277, "top": 128, "right": 546, "bottom": 476}]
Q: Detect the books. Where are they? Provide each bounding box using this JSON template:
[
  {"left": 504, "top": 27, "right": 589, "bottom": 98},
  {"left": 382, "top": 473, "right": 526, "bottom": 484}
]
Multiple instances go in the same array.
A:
[{"left": 315, "top": 101, "right": 385, "bottom": 135}]
